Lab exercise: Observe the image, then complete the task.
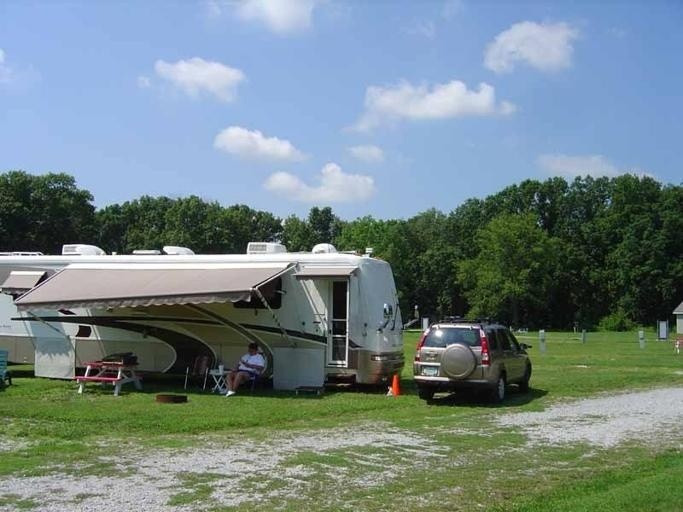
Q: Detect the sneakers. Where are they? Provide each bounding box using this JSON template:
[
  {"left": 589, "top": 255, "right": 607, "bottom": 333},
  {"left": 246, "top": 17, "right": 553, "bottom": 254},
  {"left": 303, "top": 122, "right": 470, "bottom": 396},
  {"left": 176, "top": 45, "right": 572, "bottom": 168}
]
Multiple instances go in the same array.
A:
[
  {"left": 225, "top": 391, "right": 235, "bottom": 397},
  {"left": 220, "top": 389, "right": 227, "bottom": 394}
]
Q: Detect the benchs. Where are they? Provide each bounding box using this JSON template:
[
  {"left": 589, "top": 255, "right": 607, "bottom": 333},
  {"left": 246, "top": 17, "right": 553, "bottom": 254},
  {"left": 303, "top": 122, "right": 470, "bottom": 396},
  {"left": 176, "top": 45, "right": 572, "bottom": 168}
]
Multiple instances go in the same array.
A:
[
  {"left": 74, "top": 361, "right": 143, "bottom": 396},
  {"left": 0, "top": 350, "right": 11, "bottom": 388},
  {"left": 674, "top": 336, "right": 683, "bottom": 354}
]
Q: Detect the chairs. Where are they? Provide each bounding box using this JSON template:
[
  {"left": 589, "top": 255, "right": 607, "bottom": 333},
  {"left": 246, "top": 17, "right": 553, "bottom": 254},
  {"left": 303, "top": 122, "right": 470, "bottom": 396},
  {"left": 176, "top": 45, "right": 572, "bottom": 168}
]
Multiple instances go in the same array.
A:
[{"left": 183, "top": 352, "right": 268, "bottom": 394}]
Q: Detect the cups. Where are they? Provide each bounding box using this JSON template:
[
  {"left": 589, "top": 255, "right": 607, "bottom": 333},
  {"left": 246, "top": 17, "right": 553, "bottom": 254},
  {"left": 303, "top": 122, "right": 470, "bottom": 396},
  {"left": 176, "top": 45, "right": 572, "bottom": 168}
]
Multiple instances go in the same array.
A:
[{"left": 218, "top": 365, "right": 224, "bottom": 375}]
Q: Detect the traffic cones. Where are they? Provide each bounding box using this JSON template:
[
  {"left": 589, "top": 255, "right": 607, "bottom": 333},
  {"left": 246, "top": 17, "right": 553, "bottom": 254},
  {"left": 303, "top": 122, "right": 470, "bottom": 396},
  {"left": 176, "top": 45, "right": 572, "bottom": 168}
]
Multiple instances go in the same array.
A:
[{"left": 390, "top": 373, "right": 403, "bottom": 399}]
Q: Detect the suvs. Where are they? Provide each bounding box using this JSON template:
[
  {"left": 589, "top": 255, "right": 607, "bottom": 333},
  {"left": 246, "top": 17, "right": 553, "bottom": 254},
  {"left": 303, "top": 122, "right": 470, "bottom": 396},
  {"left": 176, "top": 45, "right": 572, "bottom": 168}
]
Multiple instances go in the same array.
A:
[{"left": 409, "top": 317, "right": 536, "bottom": 409}]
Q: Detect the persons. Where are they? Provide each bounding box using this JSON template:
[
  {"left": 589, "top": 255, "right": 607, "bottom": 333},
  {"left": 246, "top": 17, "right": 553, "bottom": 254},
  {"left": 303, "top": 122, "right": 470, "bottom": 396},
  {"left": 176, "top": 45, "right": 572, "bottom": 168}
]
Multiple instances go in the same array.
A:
[{"left": 225, "top": 342, "right": 267, "bottom": 397}]
